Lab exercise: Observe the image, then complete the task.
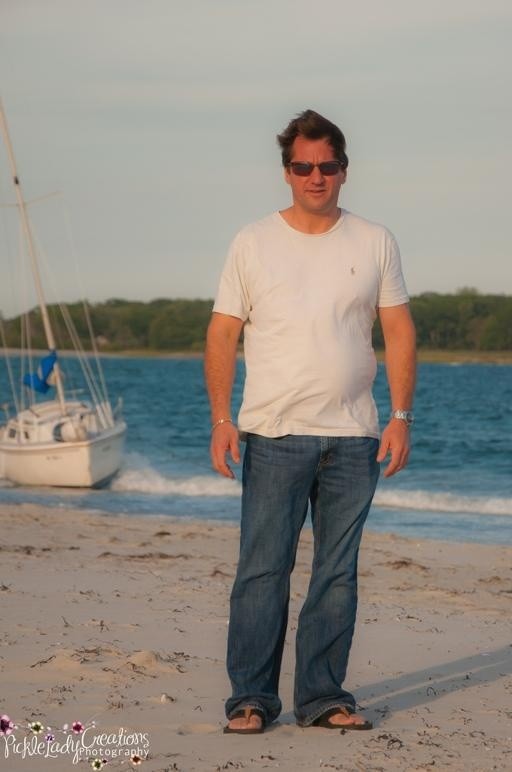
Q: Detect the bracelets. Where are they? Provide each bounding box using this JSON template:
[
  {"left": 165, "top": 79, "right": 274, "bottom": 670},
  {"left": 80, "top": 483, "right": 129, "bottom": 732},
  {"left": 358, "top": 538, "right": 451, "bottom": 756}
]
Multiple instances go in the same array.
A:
[{"left": 212, "top": 418, "right": 233, "bottom": 430}]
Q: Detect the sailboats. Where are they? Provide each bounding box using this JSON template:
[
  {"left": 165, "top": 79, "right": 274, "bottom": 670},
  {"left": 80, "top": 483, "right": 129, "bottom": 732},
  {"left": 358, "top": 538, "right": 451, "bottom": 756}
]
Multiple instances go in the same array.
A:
[{"left": 0, "top": 108, "right": 126, "bottom": 495}]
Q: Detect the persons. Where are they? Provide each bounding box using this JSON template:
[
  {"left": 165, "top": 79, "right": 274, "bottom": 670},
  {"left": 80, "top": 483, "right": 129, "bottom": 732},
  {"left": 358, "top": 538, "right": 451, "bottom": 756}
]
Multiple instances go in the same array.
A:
[{"left": 198, "top": 107, "right": 419, "bottom": 734}]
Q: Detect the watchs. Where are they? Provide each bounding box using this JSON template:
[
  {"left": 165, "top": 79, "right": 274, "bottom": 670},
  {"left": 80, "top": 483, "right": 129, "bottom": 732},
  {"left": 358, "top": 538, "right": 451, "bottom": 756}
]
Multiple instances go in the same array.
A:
[{"left": 390, "top": 408, "right": 414, "bottom": 426}]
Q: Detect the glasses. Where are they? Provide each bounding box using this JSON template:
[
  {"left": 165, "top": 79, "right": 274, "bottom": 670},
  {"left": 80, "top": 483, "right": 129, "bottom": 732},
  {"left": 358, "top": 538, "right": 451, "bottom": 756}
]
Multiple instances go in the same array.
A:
[{"left": 286, "top": 162, "right": 339, "bottom": 176}]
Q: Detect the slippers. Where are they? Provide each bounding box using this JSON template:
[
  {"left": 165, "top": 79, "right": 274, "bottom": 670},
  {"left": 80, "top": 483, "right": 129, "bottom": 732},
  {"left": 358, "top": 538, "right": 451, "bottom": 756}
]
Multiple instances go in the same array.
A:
[
  {"left": 223, "top": 708, "right": 264, "bottom": 734},
  {"left": 312, "top": 706, "right": 372, "bottom": 730}
]
